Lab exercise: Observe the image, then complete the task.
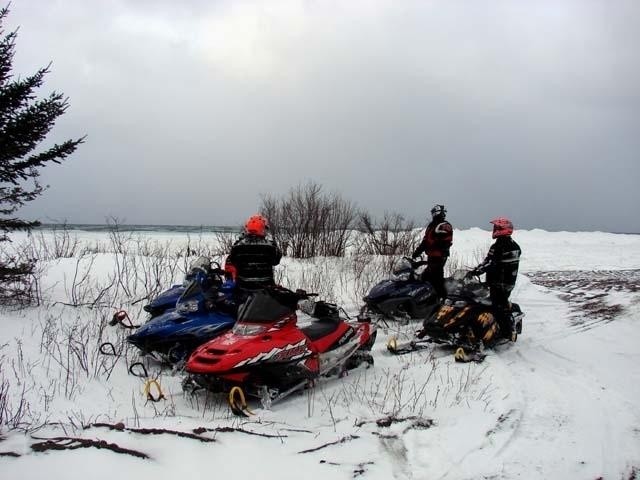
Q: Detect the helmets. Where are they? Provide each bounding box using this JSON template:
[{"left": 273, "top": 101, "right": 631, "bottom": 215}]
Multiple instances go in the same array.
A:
[
  {"left": 489, "top": 218, "right": 514, "bottom": 238},
  {"left": 246, "top": 214, "right": 269, "bottom": 238},
  {"left": 431, "top": 205, "right": 447, "bottom": 219}
]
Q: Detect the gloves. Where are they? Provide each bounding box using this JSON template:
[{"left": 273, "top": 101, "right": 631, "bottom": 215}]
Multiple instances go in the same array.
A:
[{"left": 466, "top": 271, "right": 477, "bottom": 280}]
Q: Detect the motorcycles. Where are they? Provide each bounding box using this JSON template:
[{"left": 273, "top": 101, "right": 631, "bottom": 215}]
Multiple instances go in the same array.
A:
[{"left": 358, "top": 256, "right": 525, "bottom": 364}]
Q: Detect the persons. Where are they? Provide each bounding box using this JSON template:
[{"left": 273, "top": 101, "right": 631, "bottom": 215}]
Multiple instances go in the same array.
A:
[
  {"left": 224, "top": 213, "right": 282, "bottom": 288},
  {"left": 412, "top": 203, "right": 454, "bottom": 300},
  {"left": 466, "top": 214, "right": 523, "bottom": 341}
]
